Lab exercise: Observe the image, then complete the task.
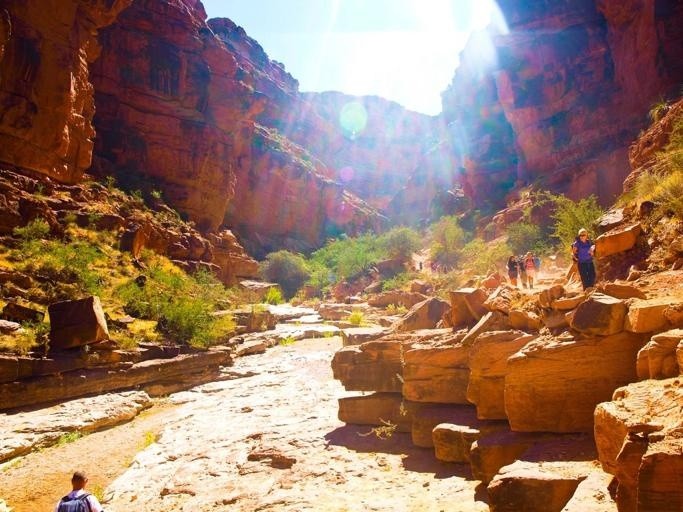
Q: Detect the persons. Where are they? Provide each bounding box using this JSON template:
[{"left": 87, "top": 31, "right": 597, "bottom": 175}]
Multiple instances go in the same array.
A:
[
  {"left": 572, "top": 228, "right": 596, "bottom": 292},
  {"left": 506, "top": 251, "right": 540, "bottom": 290},
  {"left": 431, "top": 261, "right": 448, "bottom": 274},
  {"left": 56, "top": 472, "right": 105, "bottom": 512},
  {"left": 420, "top": 261, "right": 423, "bottom": 269}
]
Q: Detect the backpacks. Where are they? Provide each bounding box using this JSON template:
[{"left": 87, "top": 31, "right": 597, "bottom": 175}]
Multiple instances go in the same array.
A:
[{"left": 58, "top": 494, "right": 90, "bottom": 512}]
